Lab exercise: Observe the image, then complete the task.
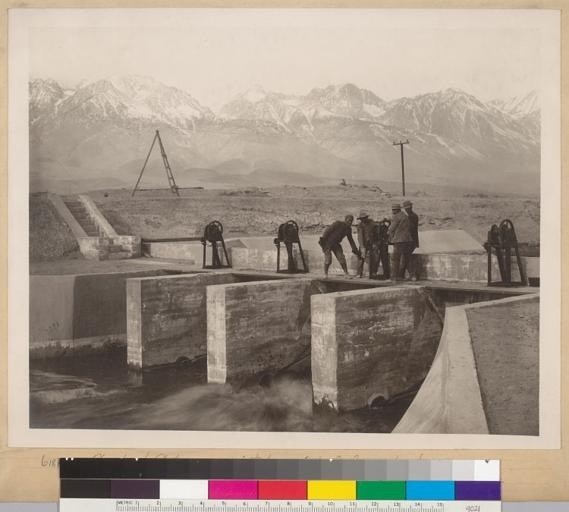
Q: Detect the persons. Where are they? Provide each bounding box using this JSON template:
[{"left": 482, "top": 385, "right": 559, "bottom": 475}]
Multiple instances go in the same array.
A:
[
  {"left": 318, "top": 215, "right": 360, "bottom": 279},
  {"left": 385, "top": 203, "right": 417, "bottom": 281},
  {"left": 353, "top": 212, "right": 380, "bottom": 279},
  {"left": 397, "top": 200, "right": 420, "bottom": 281}
]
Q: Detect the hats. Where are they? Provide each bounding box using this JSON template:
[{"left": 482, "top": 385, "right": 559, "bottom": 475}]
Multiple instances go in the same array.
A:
[
  {"left": 391, "top": 204, "right": 400, "bottom": 209},
  {"left": 357, "top": 213, "right": 370, "bottom": 220},
  {"left": 402, "top": 201, "right": 413, "bottom": 208}
]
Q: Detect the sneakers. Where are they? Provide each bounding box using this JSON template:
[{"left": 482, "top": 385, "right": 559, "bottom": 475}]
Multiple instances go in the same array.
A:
[
  {"left": 323, "top": 274, "right": 328, "bottom": 279},
  {"left": 356, "top": 274, "right": 363, "bottom": 278},
  {"left": 343, "top": 273, "right": 352, "bottom": 279},
  {"left": 390, "top": 275, "right": 417, "bottom": 281}
]
